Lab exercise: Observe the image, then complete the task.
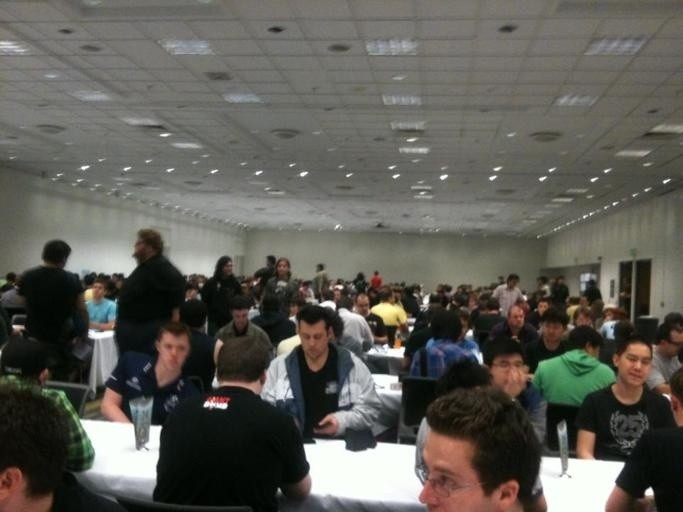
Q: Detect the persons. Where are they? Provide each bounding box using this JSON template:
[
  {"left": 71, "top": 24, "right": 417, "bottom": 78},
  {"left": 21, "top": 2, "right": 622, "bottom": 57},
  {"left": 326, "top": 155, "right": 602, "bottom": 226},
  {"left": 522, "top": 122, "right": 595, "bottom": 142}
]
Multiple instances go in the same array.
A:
[{"left": 0, "top": 226, "right": 683, "bottom": 512}]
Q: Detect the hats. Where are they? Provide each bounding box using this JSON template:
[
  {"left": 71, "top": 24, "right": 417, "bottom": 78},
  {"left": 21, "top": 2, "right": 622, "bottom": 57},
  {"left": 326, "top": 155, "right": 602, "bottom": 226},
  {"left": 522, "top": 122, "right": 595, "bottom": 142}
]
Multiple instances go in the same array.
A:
[{"left": 1, "top": 336, "right": 48, "bottom": 376}]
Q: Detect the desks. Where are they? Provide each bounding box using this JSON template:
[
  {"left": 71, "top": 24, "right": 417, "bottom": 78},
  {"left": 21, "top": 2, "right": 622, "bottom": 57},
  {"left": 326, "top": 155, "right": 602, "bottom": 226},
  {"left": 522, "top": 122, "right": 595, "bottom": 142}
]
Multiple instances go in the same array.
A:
[{"left": 67, "top": 312, "right": 681, "bottom": 508}]
